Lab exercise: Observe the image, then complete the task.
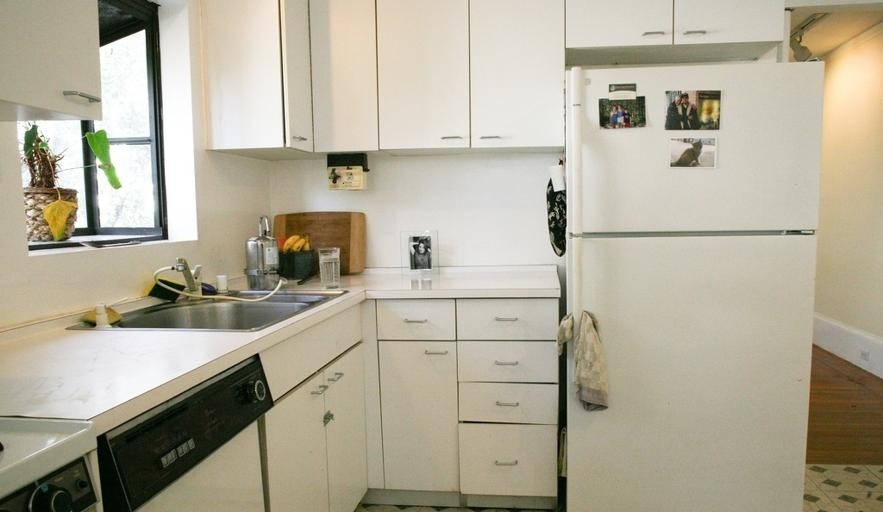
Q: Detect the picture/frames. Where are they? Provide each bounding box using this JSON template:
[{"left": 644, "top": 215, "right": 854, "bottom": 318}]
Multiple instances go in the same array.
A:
[{"left": 399, "top": 229, "right": 441, "bottom": 282}]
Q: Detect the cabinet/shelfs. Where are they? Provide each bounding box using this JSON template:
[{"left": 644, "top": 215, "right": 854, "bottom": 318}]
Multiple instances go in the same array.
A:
[
  {"left": 201, "top": 1, "right": 313, "bottom": 160},
  {"left": 0, "top": 0, "right": 103, "bottom": 120},
  {"left": 263, "top": 341, "right": 369, "bottom": 511},
  {"left": 565, "top": 1, "right": 783, "bottom": 63},
  {"left": 374, "top": 297, "right": 559, "bottom": 510},
  {"left": 374, "top": 1, "right": 565, "bottom": 148}
]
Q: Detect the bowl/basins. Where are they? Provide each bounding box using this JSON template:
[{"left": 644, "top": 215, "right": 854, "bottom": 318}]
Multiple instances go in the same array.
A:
[{"left": 280, "top": 248, "right": 319, "bottom": 279}]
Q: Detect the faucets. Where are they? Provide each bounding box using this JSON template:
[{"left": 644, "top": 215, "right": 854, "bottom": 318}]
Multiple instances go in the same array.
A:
[{"left": 172, "top": 256, "right": 197, "bottom": 294}]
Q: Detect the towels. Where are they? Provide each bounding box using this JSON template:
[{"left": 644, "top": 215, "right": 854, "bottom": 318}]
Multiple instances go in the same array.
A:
[{"left": 545, "top": 308, "right": 610, "bottom": 413}]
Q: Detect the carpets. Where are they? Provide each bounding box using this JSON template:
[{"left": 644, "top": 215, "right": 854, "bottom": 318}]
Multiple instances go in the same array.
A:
[{"left": 799, "top": 464, "right": 883, "bottom": 512}]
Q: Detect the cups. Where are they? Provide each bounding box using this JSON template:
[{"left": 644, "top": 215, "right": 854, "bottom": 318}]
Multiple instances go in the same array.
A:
[
  {"left": 216, "top": 275, "right": 228, "bottom": 293},
  {"left": 317, "top": 247, "right": 341, "bottom": 290}
]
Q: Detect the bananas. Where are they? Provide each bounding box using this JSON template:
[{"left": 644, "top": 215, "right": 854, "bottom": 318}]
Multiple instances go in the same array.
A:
[{"left": 283, "top": 233, "right": 310, "bottom": 255}]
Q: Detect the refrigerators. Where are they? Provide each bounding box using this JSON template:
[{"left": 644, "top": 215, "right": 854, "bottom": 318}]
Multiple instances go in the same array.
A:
[{"left": 562, "top": 59, "right": 827, "bottom": 512}]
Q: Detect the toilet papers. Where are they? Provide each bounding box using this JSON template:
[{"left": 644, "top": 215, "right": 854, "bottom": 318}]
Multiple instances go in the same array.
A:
[{"left": 548, "top": 164, "right": 566, "bottom": 193}]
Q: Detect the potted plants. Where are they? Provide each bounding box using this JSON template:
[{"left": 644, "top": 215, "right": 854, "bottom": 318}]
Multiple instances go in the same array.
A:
[{"left": 17, "top": 118, "right": 123, "bottom": 242}]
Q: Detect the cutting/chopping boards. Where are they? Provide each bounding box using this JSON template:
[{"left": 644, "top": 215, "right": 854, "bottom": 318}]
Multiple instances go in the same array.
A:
[{"left": 272, "top": 210, "right": 367, "bottom": 275}]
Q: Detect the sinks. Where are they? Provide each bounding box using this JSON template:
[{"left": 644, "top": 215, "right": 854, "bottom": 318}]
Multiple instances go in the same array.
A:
[
  {"left": 68, "top": 301, "right": 311, "bottom": 332},
  {"left": 231, "top": 287, "right": 348, "bottom": 305}
]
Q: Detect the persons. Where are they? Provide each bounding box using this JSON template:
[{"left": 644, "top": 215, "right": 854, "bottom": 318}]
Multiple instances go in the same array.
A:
[
  {"left": 621, "top": 108, "right": 631, "bottom": 128},
  {"left": 409, "top": 239, "right": 431, "bottom": 268},
  {"left": 681, "top": 92, "right": 698, "bottom": 129},
  {"left": 664, "top": 94, "right": 696, "bottom": 130},
  {"left": 608, "top": 105, "right": 618, "bottom": 128},
  {"left": 616, "top": 103, "right": 624, "bottom": 128}
]
